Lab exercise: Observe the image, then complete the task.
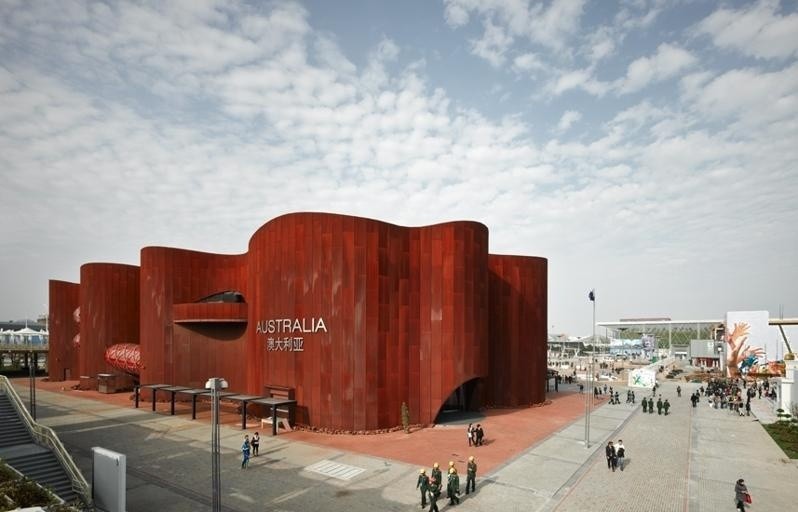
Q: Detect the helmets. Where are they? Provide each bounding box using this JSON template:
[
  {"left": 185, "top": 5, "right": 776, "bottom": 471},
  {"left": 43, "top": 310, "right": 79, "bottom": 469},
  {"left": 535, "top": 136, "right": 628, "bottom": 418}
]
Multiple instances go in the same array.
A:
[{"left": 420, "top": 456, "right": 474, "bottom": 482}]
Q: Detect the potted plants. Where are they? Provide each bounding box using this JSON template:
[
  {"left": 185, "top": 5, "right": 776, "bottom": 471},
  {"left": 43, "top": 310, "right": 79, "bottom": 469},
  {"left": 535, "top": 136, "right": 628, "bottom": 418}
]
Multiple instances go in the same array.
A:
[{"left": 0, "top": 457, "right": 93, "bottom": 512}]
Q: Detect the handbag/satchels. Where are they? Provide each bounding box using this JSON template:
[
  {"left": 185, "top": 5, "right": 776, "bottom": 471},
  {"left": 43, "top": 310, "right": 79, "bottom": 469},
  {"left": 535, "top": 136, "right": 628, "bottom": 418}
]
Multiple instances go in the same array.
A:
[{"left": 746, "top": 494, "right": 752, "bottom": 503}]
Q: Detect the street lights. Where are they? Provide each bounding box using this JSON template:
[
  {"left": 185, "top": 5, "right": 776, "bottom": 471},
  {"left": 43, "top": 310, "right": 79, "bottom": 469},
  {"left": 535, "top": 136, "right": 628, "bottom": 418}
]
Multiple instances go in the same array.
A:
[
  {"left": 204, "top": 376, "right": 228, "bottom": 512},
  {"left": 615, "top": 326, "right": 661, "bottom": 382},
  {"left": 587, "top": 287, "right": 597, "bottom": 409}
]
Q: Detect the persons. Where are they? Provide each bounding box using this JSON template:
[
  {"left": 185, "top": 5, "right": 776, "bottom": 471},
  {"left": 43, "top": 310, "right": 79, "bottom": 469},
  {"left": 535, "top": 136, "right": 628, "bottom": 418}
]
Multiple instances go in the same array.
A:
[
  {"left": 241, "top": 434, "right": 251, "bottom": 469},
  {"left": 605, "top": 442, "right": 615, "bottom": 472},
  {"left": 251, "top": 432, "right": 261, "bottom": 457},
  {"left": 416, "top": 456, "right": 477, "bottom": 512},
  {"left": 613, "top": 440, "right": 626, "bottom": 471},
  {"left": 467, "top": 423, "right": 476, "bottom": 446},
  {"left": 735, "top": 479, "right": 748, "bottom": 512},
  {"left": 552, "top": 352, "right": 777, "bottom": 416},
  {"left": 474, "top": 424, "right": 483, "bottom": 446},
  {"left": 727, "top": 319, "right": 798, "bottom": 382}
]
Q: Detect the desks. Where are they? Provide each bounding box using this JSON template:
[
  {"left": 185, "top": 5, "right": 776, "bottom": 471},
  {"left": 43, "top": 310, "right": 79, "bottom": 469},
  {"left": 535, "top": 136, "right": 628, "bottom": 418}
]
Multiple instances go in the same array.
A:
[{"left": 133, "top": 382, "right": 299, "bottom": 436}]
